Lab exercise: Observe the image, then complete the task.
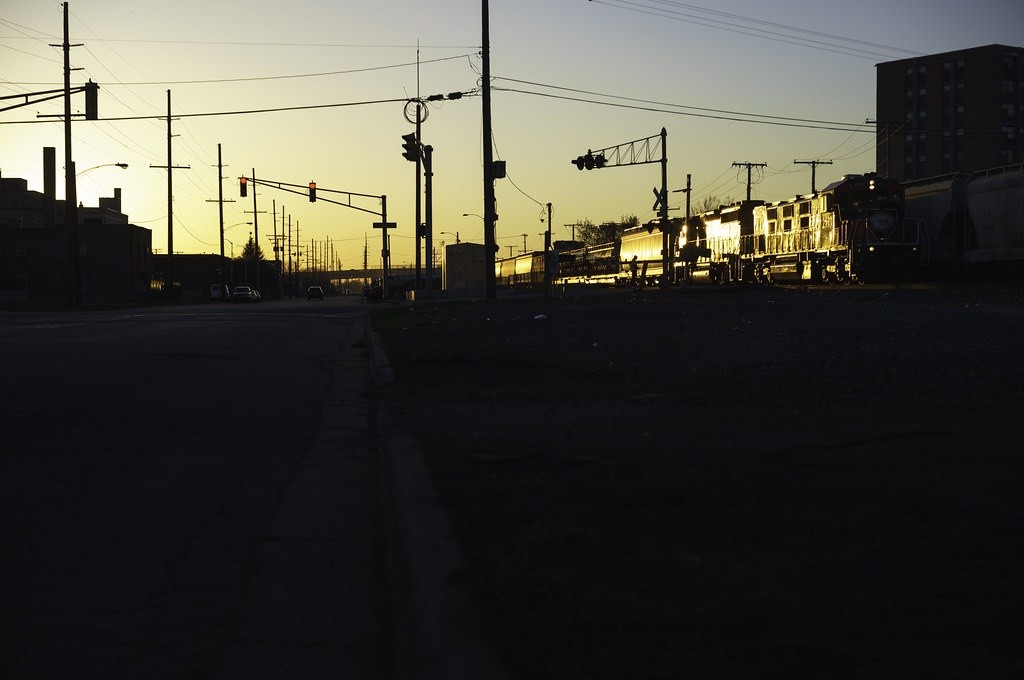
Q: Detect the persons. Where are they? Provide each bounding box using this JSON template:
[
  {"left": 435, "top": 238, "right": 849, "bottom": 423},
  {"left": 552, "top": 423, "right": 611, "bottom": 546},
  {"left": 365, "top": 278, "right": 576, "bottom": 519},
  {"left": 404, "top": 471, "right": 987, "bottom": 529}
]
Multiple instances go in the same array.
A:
[{"left": 629, "top": 256, "right": 641, "bottom": 286}]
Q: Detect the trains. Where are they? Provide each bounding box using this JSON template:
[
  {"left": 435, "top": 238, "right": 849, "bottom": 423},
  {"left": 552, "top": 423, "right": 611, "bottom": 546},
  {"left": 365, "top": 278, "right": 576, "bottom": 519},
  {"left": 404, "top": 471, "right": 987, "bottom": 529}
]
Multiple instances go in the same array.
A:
[{"left": 494, "top": 170, "right": 945, "bottom": 286}]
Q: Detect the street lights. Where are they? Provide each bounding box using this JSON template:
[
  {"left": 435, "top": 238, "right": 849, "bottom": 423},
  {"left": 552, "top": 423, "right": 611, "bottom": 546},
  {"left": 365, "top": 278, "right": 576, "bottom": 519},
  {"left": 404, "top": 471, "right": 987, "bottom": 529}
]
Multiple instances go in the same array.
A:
[{"left": 440, "top": 231, "right": 460, "bottom": 245}]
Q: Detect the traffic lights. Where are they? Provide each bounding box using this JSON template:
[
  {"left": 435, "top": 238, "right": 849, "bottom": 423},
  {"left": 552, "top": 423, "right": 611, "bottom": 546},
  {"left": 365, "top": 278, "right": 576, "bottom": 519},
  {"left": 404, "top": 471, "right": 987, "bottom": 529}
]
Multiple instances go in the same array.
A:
[
  {"left": 308, "top": 182, "right": 316, "bottom": 203},
  {"left": 240, "top": 177, "right": 247, "bottom": 198},
  {"left": 401, "top": 132, "right": 418, "bottom": 162}
]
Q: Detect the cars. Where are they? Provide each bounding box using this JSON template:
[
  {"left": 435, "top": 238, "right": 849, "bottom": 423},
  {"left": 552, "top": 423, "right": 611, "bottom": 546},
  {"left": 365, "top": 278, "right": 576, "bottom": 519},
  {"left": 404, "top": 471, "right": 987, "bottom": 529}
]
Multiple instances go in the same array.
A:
[
  {"left": 306, "top": 286, "right": 325, "bottom": 301},
  {"left": 231, "top": 286, "right": 262, "bottom": 303}
]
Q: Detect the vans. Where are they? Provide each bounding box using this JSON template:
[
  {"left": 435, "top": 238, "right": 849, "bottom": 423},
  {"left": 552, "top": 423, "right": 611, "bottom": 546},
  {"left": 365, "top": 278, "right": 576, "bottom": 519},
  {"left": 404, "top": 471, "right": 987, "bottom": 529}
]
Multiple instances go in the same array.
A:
[{"left": 208, "top": 283, "right": 230, "bottom": 300}]
